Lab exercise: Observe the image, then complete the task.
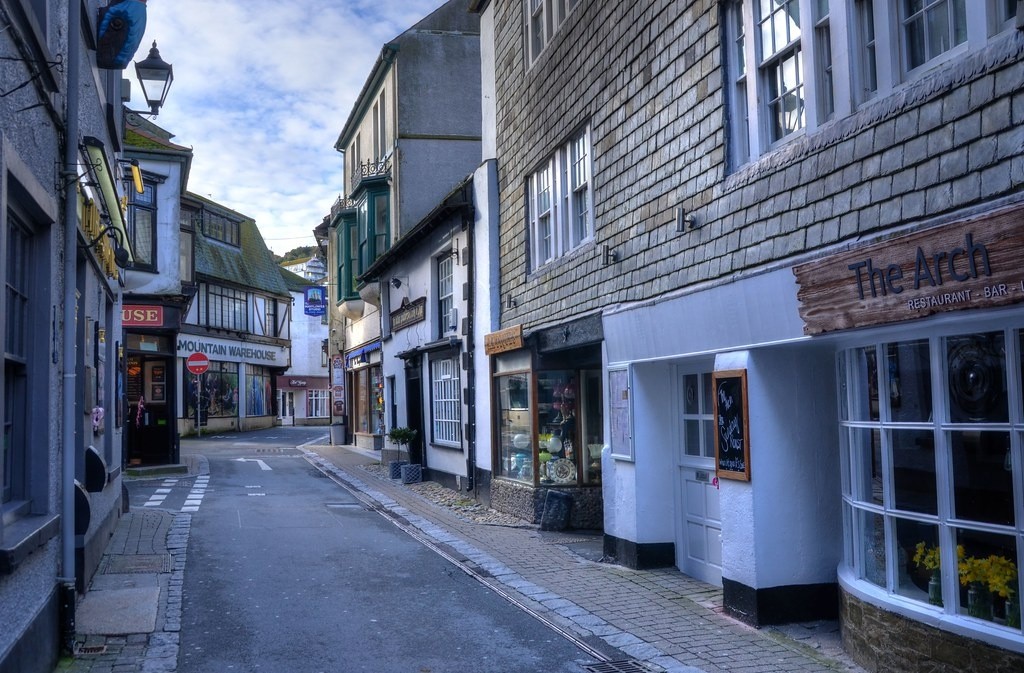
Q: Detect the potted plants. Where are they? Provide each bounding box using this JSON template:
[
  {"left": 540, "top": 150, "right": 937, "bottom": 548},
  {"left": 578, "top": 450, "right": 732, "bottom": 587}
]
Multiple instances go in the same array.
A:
[{"left": 384, "top": 426, "right": 422, "bottom": 484}]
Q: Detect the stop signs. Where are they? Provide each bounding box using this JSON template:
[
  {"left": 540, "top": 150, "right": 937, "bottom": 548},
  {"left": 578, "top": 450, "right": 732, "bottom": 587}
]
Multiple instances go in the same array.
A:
[{"left": 186, "top": 352, "right": 209, "bottom": 375}]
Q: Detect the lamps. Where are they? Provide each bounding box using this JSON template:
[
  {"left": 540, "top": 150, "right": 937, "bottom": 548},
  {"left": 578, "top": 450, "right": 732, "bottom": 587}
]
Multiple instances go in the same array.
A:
[
  {"left": 114, "top": 158, "right": 144, "bottom": 194},
  {"left": 675, "top": 208, "right": 695, "bottom": 232},
  {"left": 122, "top": 39, "right": 174, "bottom": 140},
  {"left": 602, "top": 245, "right": 617, "bottom": 265},
  {"left": 505, "top": 294, "right": 516, "bottom": 308},
  {"left": 391, "top": 272, "right": 409, "bottom": 290}
]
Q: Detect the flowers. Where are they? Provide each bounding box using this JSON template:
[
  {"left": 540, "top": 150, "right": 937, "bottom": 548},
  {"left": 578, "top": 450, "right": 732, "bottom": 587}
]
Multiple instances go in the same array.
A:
[{"left": 913, "top": 541, "right": 1018, "bottom": 601}]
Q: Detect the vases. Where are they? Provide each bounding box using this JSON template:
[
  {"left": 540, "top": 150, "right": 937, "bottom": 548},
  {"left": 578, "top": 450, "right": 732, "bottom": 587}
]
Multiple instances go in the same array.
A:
[
  {"left": 967, "top": 585, "right": 994, "bottom": 621},
  {"left": 1005, "top": 597, "right": 1021, "bottom": 630},
  {"left": 928, "top": 576, "right": 941, "bottom": 607}
]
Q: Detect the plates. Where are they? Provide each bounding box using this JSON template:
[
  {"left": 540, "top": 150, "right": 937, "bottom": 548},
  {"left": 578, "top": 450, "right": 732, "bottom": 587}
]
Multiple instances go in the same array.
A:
[{"left": 549, "top": 458, "right": 576, "bottom": 483}]
